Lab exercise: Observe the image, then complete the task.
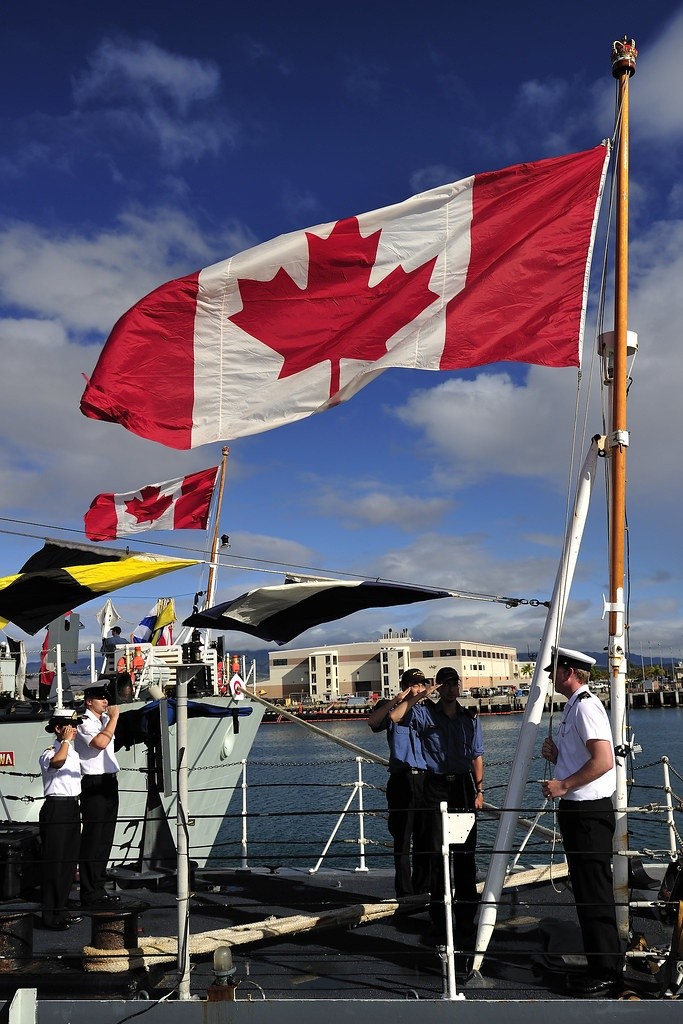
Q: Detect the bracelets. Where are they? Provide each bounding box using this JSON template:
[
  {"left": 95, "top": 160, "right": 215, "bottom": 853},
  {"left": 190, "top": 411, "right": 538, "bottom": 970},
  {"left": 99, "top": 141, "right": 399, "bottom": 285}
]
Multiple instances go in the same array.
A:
[
  {"left": 61, "top": 740, "right": 70, "bottom": 746},
  {"left": 477, "top": 789, "right": 485, "bottom": 794}
]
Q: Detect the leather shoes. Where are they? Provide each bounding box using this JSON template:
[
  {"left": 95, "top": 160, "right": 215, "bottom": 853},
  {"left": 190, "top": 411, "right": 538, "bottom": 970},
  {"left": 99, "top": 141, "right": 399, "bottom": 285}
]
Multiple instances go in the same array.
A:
[
  {"left": 98, "top": 894, "right": 121, "bottom": 903},
  {"left": 55, "top": 922, "right": 71, "bottom": 931},
  {"left": 64, "top": 915, "right": 84, "bottom": 924}
]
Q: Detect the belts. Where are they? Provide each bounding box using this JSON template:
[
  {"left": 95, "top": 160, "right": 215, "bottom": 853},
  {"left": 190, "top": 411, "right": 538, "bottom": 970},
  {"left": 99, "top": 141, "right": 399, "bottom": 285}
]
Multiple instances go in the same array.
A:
[
  {"left": 434, "top": 774, "right": 468, "bottom": 781},
  {"left": 52, "top": 795, "right": 79, "bottom": 800},
  {"left": 401, "top": 768, "right": 429, "bottom": 776}
]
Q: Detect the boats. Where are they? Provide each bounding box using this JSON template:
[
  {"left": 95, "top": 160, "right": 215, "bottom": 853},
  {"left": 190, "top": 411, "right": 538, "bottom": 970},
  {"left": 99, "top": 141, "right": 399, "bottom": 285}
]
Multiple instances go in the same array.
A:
[{"left": 0, "top": 534, "right": 270, "bottom": 869}]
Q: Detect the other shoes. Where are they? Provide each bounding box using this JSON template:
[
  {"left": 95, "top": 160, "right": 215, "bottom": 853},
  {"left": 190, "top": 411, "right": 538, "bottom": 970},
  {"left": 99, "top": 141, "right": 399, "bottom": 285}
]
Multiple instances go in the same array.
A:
[{"left": 571, "top": 976, "right": 621, "bottom": 998}]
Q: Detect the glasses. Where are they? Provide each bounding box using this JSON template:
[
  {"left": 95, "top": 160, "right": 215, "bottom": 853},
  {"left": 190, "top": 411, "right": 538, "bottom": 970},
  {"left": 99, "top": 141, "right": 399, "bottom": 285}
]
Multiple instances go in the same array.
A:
[{"left": 442, "top": 683, "right": 461, "bottom": 688}]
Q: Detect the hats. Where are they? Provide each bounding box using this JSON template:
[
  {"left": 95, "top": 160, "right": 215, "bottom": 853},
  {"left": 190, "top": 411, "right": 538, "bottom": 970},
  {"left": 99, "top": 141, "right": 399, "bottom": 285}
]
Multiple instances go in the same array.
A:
[
  {"left": 110, "top": 626, "right": 122, "bottom": 632},
  {"left": 401, "top": 668, "right": 430, "bottom": 686},
  {"left": 543, "top": 645, "right": 597, "bottom": 672},
  {"left": 84, "top": 679, "right": 111, "bottom": 698},
  {"left": 51, "top": 708, "right": 83, "bottom": 726},
  {"left": 435, "top": 667, "right": 463, "bottom": 684}
]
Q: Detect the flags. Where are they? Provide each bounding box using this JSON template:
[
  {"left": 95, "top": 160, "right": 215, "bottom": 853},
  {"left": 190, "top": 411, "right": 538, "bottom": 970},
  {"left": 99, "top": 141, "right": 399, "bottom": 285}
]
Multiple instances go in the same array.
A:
[
  {"left": 40, "top": 630, "right": 57, "bottom": 685},
  {"left": 179, "top": 570, "right": 457, "bottom": 647},
  {"left": 82, "top": 463, "right": 221, "bottom": 543},
  {"left": 0, "top": 538, "right": 206, "bottom": 636},
  {"left": 79, "top": 137, "right": 614, "bottom": 452},
  {"left": 4, "top": 636, "right": 27, "bottom": 702},
  {"left": 132, "top": 596, "right": 176, "bottom": 645}
]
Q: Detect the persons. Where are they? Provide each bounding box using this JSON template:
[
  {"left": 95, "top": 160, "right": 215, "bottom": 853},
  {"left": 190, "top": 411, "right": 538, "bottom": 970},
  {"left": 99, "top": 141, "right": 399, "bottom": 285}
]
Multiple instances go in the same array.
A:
[
  {"left": 75, "top": 679, "right": 122, "bottom": 907},
  {"left": 541, "top": 644, "right": 627, "bottom": 999},
  {"left": 389, "top": 667, "right": 485, "bottom": 941},
  {"left": 368, "top": 669, "right": 429, "bottom": 901},
  {"left": 39, "top": 708, "right": 85, "bottom": 931},
  {"left": 100, "top": 626, "right": 131, "bottom": 671}
]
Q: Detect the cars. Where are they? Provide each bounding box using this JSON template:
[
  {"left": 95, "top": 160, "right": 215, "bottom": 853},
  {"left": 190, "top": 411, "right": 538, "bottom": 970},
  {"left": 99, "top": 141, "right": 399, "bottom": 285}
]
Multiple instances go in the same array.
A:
[
  {"left": 592, "top": 678, "right": 640, "bottom": 689},
  {"left": 461, "top": 682, "right": 530, "bottom": 698},
  {"left": 346, "top": 696, "right": 367, "bottom": 709}
]
